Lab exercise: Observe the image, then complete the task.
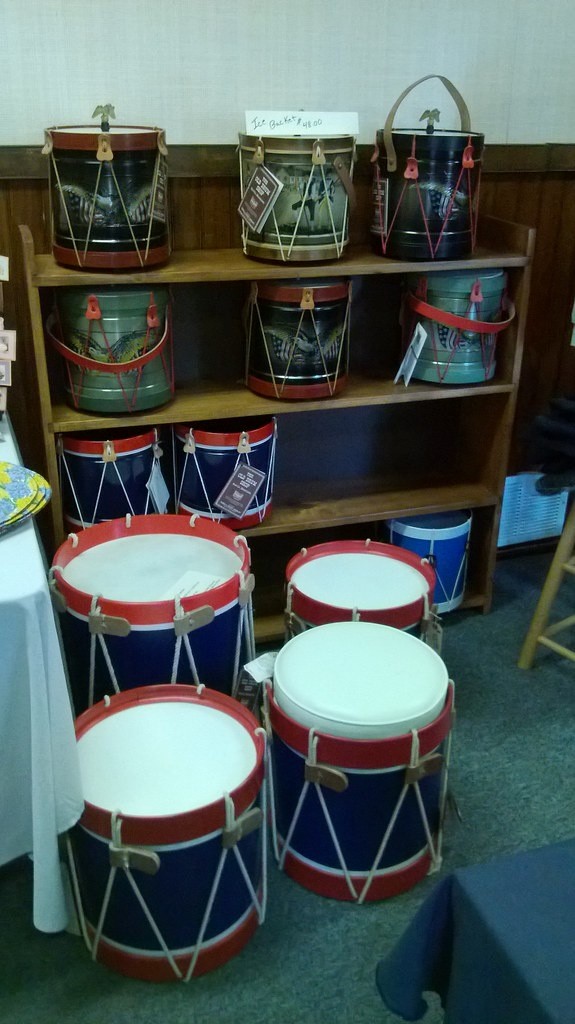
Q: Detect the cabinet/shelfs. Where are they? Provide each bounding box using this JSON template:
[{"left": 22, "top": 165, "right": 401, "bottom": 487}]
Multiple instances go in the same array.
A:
[{"left": 18, "top": 213, "right": 536, "bottom": 644}]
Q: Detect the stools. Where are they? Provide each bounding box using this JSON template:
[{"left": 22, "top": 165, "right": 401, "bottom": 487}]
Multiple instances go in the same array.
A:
[{"left": 518, "top": 498, "right": 575, "bottom": 670}]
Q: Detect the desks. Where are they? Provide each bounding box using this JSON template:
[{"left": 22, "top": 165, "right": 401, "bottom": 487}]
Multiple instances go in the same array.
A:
[
  {"left": 375, "top": 838, "right": 575, "bottom": 1024},
  {"left": 0, "top": 412, "right": 85, "bottom": 933}
]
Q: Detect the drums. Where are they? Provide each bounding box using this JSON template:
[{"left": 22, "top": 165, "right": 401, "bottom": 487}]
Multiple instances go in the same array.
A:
[
  {"left": 400, "top": 270, "right": 508, "bottom": 386},
  {"left": 45, "top": 120, "right": 171, "bottom": 271},
  {"left": 386, "top": 510, "right": 474, "bottom": 615},
  {"left": 67, "top": 681, "right": 271, "bottom": 986},
  {"left": 54, "top": 288, "right": 178, "bottom": 414},
  {"left": 280, "top": 536, "right": 438, "bottom": 650},
  {"left": 371, "top": 127, "right": 486, "bottom": 260},
  {"left": 172, "top": 415, "right": 282, "bottom": 531},
  {"left": 56, "top": 427, "right": 162, "bottom": 535},
  {"left": 47, "top": 510, "right": 255, "bottom": 718},
  {"left": 265, "top": 619, "right": 456, "bottom": 907},
  {"left": 244, "top": 281, "right": 353, "bottom": 399},
  {"left": 237, "top": 131, "right": 356, "bottom": 263}
]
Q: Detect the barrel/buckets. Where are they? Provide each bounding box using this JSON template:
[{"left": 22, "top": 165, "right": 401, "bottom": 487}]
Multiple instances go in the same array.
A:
[
  {"left": 170, "top": 414, "right": 279, "bottom": 529},
  {"left": 371, "top": 74, "right": 487, "bottom": 261},
  {"left": 400, "top": 268, "right": 516, "bottom": 385},
  {"left": 239, "top": 277, "right": 355, "bottom": 399},
  {"left": 49, "top": 513, "right": 256, "bottom": 719},
  {"left": 63, "top": 683, "right": 271, "bottom": 981},
  {"left": 234, "top": 130, "right": 360, "bottom": 261},
  {"left": 282, "top": 539, "right": 438, "bottom": 648},
  {"left": 262, "top": 620, "right": 458, "bottom": 904},
  {"left": 385, "top": 508, "right": 473, "bottom": 614},
  {"left": 39, "top": 103, "right": 174, "bottom": 269},
  {"left": 56, "top": 427, "right": 164, "bottom": 535},
  {"left": 45, "top": 286, "right": 178, "bottom": 415}
]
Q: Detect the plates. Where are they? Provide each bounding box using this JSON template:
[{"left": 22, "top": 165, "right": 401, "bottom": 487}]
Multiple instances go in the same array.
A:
[{"left": 0, "top": 460, "right": 52, "bottom": 536}]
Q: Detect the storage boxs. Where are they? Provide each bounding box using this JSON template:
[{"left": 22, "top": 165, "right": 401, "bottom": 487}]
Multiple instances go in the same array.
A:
[{"left": 271, "top": 404, "right": 383, "bottom": 487}]
